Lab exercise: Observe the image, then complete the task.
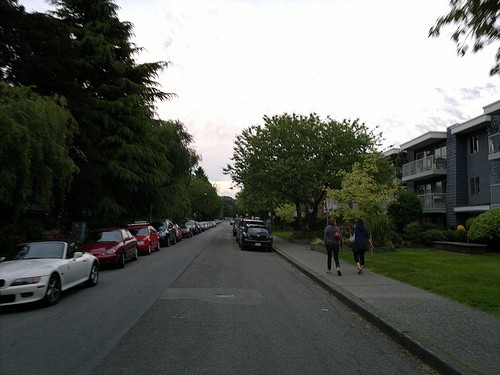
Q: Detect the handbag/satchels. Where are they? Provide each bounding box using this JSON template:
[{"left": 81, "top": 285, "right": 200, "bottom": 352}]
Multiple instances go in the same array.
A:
[
  {"left": 349, "top": 225, "right": 356, "bottom": 244},
  {"left": 335, "top": 228, "right": 341, "bottom": 241}
]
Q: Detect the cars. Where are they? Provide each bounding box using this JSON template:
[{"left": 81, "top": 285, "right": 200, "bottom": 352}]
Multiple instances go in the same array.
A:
[
  {"left": 79, "top": 228, "right": 139, "bottom": 268},
  {"left": 126, "top": 220, "right": 160, "bottom": 255},
  {"left": 149, "top": 218, "right": 223, "bottom": 247},
  {"left": 230, "top": 217, "right": 273, "bottom": 252}
]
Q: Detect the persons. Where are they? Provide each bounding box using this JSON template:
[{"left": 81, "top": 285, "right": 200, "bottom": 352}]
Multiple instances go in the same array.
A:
[
  {"left": 351, "top": 219, "right": 374, "bottom": 274},
  {"left": 324, "top": 219, "right": 342, "bottom": 276}
]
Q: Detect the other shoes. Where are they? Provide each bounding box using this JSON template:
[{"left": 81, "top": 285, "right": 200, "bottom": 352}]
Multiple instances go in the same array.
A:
[
  {"left": 337, "top": 271, "right": 342, "bottom": 276},
  {"left": 358, "top": 269, "right": 361, "bottom": 274},
  {"left": 327, "top": 271, "right": 332, "bottom": 274}
]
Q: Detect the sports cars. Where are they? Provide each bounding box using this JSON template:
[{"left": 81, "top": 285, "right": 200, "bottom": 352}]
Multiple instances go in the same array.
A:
[{"left": 0, "top": 241, "right": 100, "bottom": 307}]
